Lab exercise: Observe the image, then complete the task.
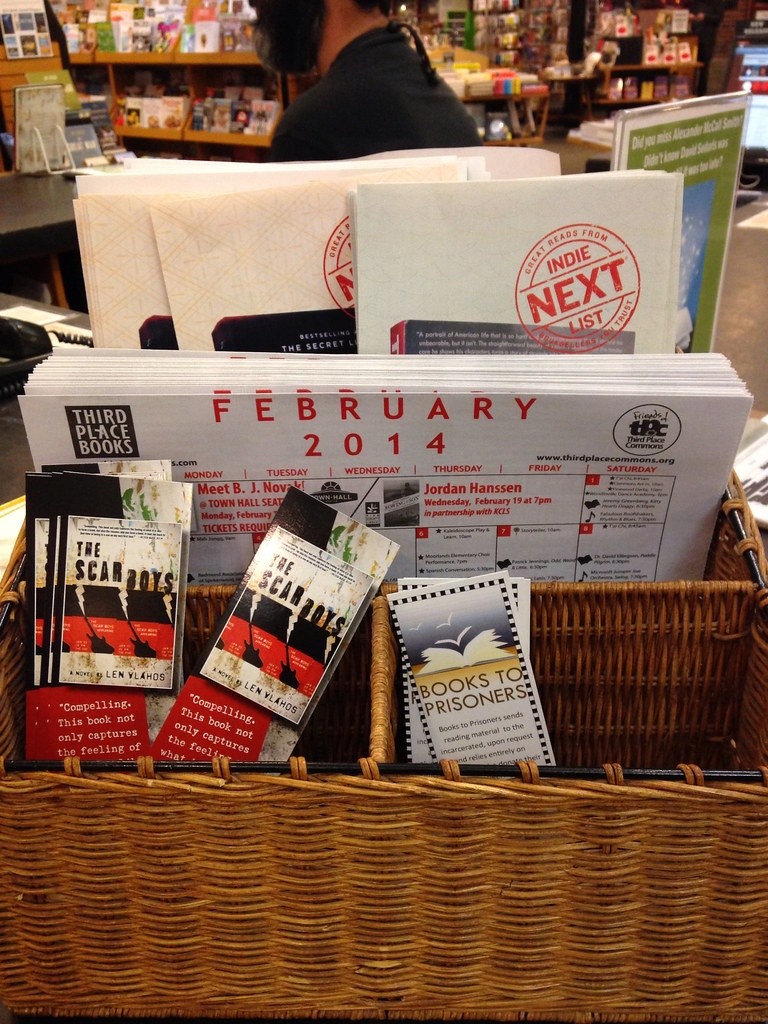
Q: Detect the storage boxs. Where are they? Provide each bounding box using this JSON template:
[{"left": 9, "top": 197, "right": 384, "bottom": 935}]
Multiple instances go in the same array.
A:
[{"left": 0, "top": 468, "right": 768, "bottom": 1024}]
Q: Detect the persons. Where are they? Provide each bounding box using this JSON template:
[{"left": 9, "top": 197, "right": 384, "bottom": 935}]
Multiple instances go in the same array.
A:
[{"left": 248, "top": 0, "right": 482, "bottom": 161}]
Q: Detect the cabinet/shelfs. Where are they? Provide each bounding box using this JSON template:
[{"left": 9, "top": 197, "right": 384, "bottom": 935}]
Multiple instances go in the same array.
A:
[{"left": 0, "top": 0, "right": 768, "bottom": 412}]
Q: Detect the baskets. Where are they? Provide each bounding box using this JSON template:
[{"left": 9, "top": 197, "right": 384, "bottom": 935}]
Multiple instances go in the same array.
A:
[{"left": 1, "top": 468, "right": 768, "bottom": 1022}]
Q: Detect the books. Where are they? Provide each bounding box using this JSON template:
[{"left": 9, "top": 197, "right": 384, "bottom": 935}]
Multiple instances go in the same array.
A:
[{"left": 1, "top": 60, "right": 753, "bottom": 767}]
